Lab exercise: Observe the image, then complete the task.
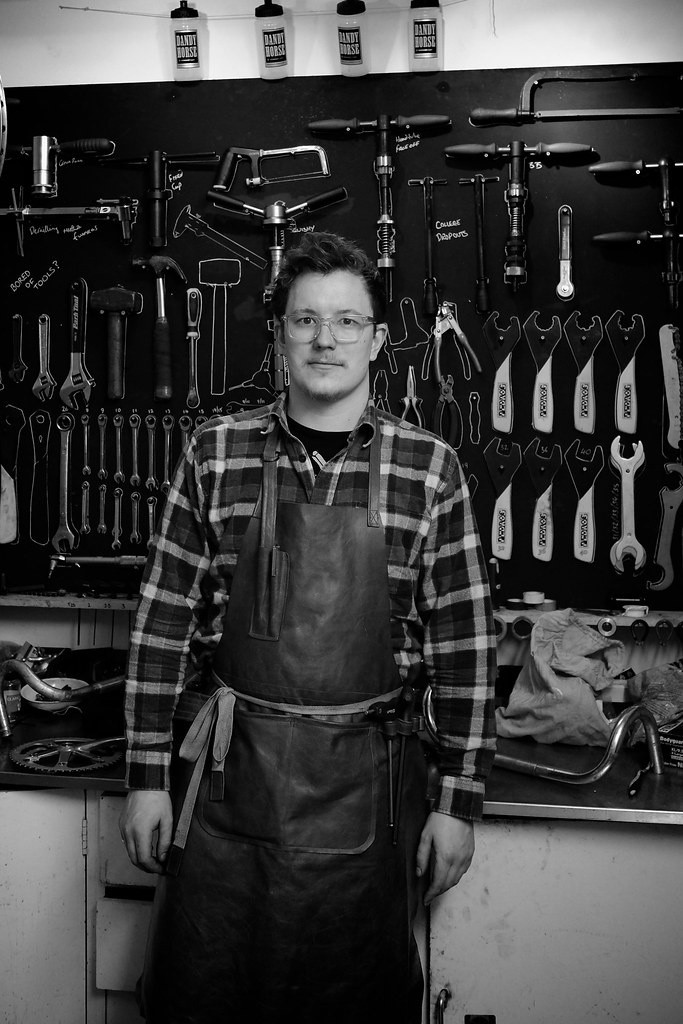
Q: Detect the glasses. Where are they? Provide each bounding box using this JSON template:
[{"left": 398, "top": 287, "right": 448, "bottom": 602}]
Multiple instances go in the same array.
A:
[{"left": 280, "top": 313, "right": 377, "bottom": 343}]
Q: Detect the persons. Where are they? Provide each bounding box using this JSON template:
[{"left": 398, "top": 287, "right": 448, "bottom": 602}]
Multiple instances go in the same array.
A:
[{"left": 123, "top": 232, "right": 499, "bottom": 1024}]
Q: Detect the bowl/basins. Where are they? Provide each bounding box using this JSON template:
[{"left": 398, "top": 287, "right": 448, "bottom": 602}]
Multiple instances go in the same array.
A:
[{"left": 21, "top": 677, "right": 89, "bottom": 710}]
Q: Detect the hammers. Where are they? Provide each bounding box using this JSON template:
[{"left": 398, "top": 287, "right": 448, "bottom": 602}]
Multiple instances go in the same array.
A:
[
  {"left": 88, "top": 283, "right": 143, "bottom": 402},
  {"left": 131, "top": 251, "right": 187, "bottom": 400}
]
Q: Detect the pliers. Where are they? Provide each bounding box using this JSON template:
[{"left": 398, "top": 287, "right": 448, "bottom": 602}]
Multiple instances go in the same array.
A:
[
  {"left": 399, "top": 366, "right": 425, "bottom": 427},
  {"left": 428, "top": 302, "right": 487, "bottom": 382},
  {"left": 433, "top": 373, "right": 464, "bottom": 450}
]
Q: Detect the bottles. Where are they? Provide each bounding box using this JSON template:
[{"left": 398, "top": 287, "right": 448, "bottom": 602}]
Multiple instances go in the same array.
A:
[
  {"left": 170, "top": 0, "right": 200, "bottom": 81},
  {"left": 2, "top": 674, "right": 20, "bottom": 723},
  {"left": 409, "top": 0, "right": 444, "bottom": 72},
  {"left": 337, "top": 0, "right": 370, "bottom": 77},
  {"left": 255, "top": 0, "right": 287, "bottom": 80}
]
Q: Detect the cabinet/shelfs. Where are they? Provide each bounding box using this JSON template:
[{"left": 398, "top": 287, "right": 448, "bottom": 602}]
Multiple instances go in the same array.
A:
[
  {"left": 93, "top": 792, "right": 683, "bottom": 1024},
  {"left": 0, "top": 787, "right": 88, "bottom": 1024}
]
